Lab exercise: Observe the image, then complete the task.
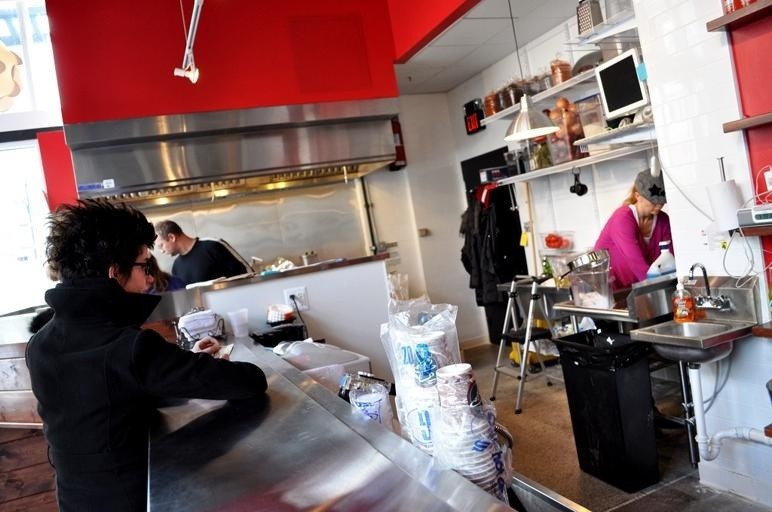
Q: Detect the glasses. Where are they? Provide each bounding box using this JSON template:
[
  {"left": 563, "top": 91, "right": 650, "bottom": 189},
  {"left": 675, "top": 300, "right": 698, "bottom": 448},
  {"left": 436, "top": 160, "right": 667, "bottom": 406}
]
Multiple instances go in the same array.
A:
[{"left": 133, "top": 261, "right": 150, "bottom": 275}]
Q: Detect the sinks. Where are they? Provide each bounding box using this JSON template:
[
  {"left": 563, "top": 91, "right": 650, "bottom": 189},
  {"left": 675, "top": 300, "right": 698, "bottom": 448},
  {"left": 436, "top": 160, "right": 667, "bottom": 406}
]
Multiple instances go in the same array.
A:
[{"left": 631, "top": 315, "right": 757, "bottom": 361}]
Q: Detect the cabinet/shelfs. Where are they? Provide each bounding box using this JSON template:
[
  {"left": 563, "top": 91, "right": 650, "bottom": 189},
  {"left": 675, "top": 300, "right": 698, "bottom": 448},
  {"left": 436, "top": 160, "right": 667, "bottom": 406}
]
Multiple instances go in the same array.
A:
[
  {"left": 705, "top": 0, "right": 772, "bottom": 236},
  {"left": 479, "top": 45, "right": 659, "bottom": 187}
]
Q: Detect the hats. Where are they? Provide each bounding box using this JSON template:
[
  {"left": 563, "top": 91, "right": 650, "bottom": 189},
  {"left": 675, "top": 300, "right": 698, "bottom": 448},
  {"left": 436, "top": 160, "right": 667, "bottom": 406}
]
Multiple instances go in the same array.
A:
[{"left": 635, "top": 169, "right": 667, "bottom": 204}]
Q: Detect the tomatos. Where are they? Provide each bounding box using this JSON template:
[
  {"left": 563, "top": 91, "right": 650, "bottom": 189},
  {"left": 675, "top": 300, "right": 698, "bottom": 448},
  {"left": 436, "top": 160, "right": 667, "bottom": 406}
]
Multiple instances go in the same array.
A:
[{"left": 545, "top": 234, "right": 569, "bottom": 248}]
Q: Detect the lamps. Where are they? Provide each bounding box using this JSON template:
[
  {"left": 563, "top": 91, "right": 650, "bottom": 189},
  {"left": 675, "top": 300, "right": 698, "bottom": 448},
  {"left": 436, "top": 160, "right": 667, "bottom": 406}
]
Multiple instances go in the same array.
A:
[
  {"left": 506, "top": 0, "right": 560, "bottom": 141},
  {"left": 174, "top": 0, "right": 204, "bottom": 83}
]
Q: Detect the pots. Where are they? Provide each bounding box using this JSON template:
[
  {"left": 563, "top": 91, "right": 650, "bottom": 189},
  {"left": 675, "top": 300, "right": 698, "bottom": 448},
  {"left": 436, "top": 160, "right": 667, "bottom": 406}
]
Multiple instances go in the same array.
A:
[{"left": 560, "top": 250, "right": 608, "bottom": 281}]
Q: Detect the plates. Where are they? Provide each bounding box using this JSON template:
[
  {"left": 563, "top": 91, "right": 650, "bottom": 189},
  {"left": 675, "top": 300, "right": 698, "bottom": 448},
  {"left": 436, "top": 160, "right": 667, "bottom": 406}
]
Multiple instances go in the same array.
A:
[{"left": 267, "top": 304, "right": 294, "bottom": 323}]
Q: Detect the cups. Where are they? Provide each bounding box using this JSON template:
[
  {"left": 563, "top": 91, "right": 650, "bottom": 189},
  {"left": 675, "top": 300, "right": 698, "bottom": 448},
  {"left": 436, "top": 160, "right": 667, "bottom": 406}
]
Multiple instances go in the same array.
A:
[
  {"left": 392, "top": 328, "right": 510, "bottom": 508},
  {"left": 350, "top": 386, "right": 391, "bottom": 428},
  {"left": 228, "top": 308, "right": 249, "bottom": 337}
]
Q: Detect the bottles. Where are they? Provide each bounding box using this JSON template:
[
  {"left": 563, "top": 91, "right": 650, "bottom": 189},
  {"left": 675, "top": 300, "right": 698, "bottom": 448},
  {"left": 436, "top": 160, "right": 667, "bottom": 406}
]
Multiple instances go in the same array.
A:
[
  {"left": 415, "top": 300, "right": 432, "bottom": 325},
  {"left": 299, "top": 250, "right": 318, "bottom": 266}
]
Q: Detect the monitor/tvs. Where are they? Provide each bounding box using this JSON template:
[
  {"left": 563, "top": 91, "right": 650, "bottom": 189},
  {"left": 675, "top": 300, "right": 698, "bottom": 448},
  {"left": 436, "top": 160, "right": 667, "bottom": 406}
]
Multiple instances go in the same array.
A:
[{"left": 594, "top": 47, "right": 650, "bottom": 121}]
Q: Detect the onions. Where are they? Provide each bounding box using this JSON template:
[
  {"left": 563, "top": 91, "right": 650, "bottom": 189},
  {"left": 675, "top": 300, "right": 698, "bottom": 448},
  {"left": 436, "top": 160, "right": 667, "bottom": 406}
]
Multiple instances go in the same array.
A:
[{"left": 549, "top": 97, "right": 582, "bottom": 139}]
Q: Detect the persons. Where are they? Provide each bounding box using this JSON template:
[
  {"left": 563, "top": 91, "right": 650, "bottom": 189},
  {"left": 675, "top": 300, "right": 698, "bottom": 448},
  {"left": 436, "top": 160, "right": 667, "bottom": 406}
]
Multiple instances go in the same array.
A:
[
  {"left": 26, "top": 198, "right": 268, "bottom": 512},
  {"left": 593, "top": 170, "right": 674, "bottom": 329},
  {"left": 142, "top": 255, "right": 186, "bottom": 295},
  {"left": 153, "top": 221, "right": 255, "bottom": 285}
]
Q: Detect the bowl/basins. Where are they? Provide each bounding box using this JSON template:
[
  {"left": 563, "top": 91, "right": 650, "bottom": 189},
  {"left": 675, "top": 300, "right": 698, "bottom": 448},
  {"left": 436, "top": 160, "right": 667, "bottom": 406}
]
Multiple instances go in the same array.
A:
[{"left": 539, "top": 231, "right": 575, "bottom": 251}]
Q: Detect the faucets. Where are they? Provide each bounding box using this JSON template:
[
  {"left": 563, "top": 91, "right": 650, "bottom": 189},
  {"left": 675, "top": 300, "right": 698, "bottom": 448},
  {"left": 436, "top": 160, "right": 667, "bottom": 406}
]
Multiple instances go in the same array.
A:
[{"left": 689, "top": 262, "right": 711, "bottom": 298}]
